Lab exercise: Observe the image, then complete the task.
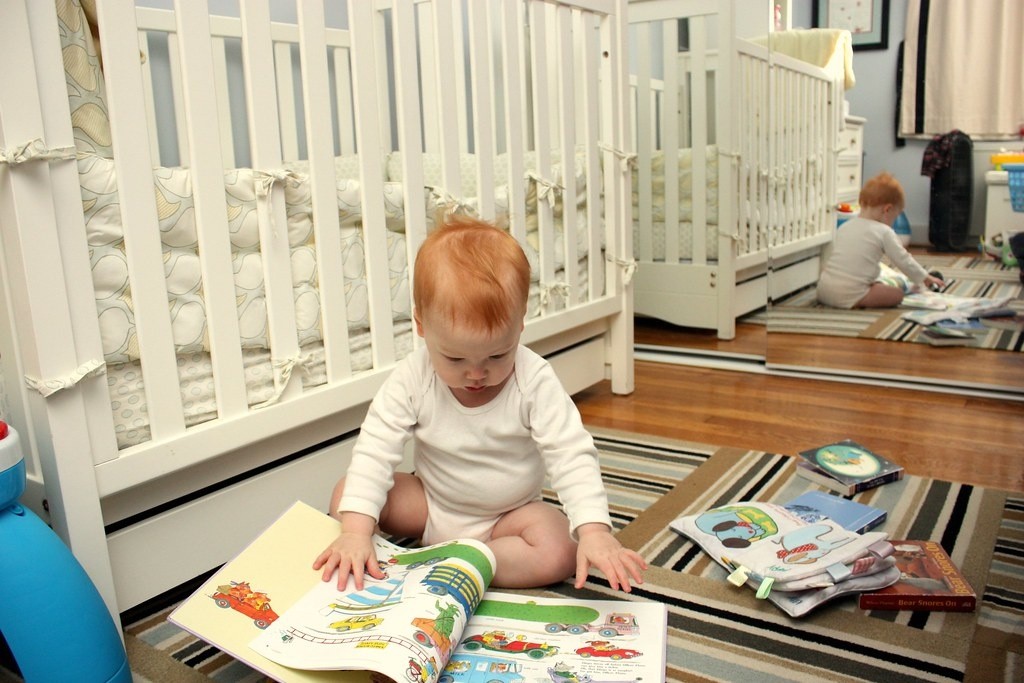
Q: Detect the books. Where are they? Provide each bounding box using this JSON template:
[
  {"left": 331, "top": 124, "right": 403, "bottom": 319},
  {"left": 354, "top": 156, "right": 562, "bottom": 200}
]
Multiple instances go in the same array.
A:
[
  {"left": 783, "top": 491, "right": 887, "bottom": 534},
  {"left": 796, "top": 439, "right": 905, "bottom": 496},
  {"left": 921, "top": 317, "right": 1024, "bottom": 346},
  {"left": 166, "top": 499, "right": 667, "bottom": 683},
  {"left": 855, "top": 540, "right": 977, "bottom": 611}
]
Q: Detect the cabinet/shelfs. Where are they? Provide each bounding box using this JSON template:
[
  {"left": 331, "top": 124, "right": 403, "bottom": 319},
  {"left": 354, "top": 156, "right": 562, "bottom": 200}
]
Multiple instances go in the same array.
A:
[
  {"left": 837, "top": 114, "right": 866, "bottom": 209},
  {"left": 982, "top": 170, "right": 1024, "bottom": 246}
]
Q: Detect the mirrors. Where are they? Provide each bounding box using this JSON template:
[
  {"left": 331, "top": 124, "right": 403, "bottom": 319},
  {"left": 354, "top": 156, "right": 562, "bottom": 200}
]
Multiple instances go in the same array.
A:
[{"left": 630, "top": 0, "right": 1024, "bottom": 403}]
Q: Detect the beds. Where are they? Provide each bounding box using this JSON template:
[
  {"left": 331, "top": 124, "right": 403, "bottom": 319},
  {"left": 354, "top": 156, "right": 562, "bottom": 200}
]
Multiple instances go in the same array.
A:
[
  {"left": 629, "top": 1, "right": 854, "bottom": 303},
  {"left": 0, "top": 1, "right": 630, "bottom": 655}
]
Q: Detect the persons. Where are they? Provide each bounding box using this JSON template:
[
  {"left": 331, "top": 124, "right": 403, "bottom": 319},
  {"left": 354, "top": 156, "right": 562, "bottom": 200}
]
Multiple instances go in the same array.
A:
[
  {"left": 314, "top": 222, "right": 648, "bottom": 591},
  {"left": 815, "top": 172, "right": 947, "bottom": 308}
]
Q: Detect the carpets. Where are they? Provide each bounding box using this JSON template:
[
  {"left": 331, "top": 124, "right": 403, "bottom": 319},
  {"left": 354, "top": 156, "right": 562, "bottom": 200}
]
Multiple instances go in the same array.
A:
[
  {"left": 742, "top": 256, "right": 1024, "bottom": 353},
  {"left": 119, "top": 420, "right": 1023, "bottom": 683}
]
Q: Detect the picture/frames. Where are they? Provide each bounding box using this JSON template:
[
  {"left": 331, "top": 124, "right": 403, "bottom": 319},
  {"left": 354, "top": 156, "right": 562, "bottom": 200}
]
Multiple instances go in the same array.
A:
[{"left": 811, "top": 0, "right": 889, "bottom": 51}]
